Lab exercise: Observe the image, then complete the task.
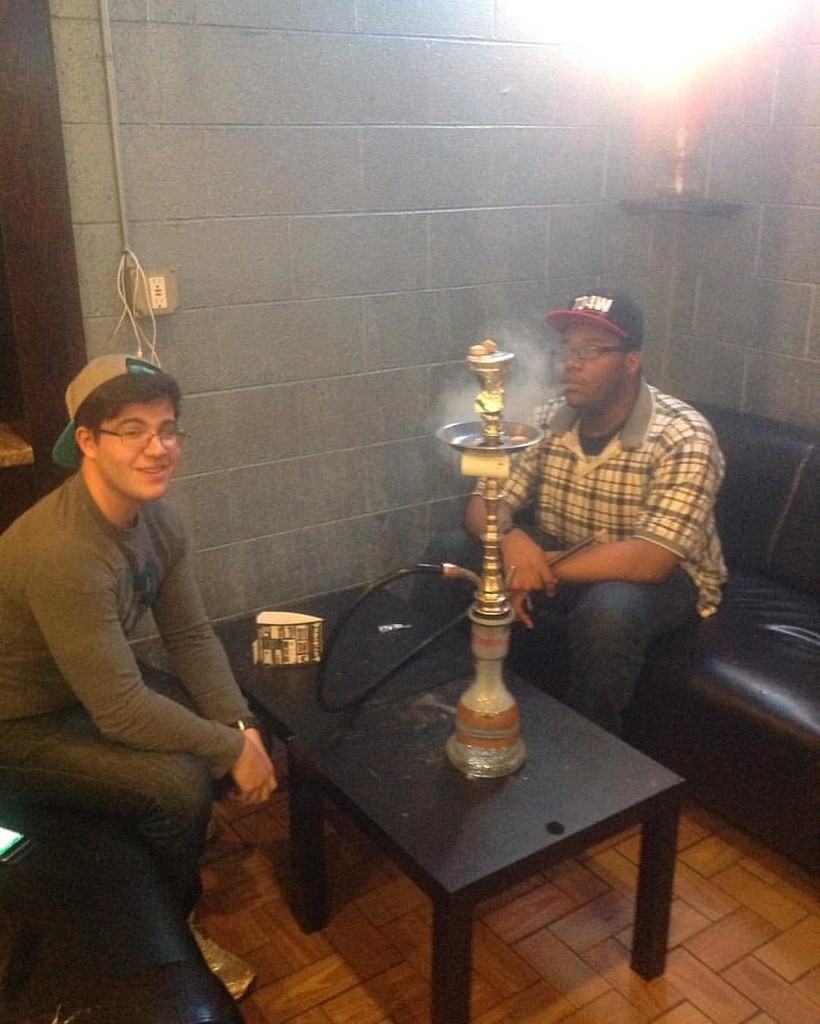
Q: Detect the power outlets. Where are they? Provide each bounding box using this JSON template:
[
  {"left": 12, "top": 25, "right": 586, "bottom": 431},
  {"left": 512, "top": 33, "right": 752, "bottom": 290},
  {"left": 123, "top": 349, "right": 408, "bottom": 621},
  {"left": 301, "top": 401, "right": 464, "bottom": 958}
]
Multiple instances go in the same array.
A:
[{"left": 128, "top": 268, "right": 181, "bottom": 317}]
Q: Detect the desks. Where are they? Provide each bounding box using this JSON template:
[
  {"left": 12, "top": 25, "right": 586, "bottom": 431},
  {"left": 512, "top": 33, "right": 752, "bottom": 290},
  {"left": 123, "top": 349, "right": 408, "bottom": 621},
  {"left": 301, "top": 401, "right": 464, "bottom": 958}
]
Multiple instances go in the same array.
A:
[
  {"left": 287, "top": 667, "right": 686, "bottom": 1024},
  {"left": 214, "top": 580, "right": 476, "bottom": 742}
]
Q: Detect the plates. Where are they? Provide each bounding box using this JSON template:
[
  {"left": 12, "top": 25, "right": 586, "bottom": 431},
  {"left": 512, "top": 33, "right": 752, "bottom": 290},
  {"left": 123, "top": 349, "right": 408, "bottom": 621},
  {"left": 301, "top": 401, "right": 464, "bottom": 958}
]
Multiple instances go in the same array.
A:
[{"left": 436, "top": 419, "right": 544, "bottom": 457}]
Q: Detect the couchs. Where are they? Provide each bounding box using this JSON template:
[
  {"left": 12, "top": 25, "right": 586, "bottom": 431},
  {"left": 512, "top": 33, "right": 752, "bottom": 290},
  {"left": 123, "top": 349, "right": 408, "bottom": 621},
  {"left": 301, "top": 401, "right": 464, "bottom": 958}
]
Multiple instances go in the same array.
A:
[
  {"left": 0, "top": 793, "right": 245, "bottom": 1024},
  {"left": 508, "top": 398, "right": 820, "bottom": 876}
]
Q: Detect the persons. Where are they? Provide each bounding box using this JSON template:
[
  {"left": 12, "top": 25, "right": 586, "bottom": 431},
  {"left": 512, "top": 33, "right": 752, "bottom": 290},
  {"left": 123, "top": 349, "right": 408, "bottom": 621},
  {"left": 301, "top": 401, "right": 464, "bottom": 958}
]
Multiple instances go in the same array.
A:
[
  {"left": 0, "top": 353, "right": 277, "bottom": 1001},
  {"left": 463, "top": 290, "right": 728, "bottom": 733}
]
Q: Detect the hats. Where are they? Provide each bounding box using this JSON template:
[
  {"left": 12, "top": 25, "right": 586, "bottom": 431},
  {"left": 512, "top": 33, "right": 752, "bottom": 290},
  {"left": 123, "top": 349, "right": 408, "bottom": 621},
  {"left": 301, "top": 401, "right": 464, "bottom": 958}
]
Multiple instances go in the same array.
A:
[
  {"left": 546, "top": 288, "right": 644, "bottom": 349},
  {"left": 51, "top": 354, "right": 168, "bottom": 468}
]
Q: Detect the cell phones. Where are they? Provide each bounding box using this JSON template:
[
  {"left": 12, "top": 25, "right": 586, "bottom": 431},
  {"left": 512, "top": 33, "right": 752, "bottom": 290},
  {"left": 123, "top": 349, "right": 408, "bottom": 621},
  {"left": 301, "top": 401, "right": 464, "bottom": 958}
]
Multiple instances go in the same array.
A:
[{"left": 0, "top": 826, "right": 31, "bottom": 862}]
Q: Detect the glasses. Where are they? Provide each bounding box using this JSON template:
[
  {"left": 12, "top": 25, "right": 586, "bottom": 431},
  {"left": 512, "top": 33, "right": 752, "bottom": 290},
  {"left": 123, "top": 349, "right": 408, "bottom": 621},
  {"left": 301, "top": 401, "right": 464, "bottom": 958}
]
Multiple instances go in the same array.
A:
[
  {"left": 96, "top": 425, "right": 191, "bottom": 450},
  {"left": 555, "top": 346, "right": 625, "bottom": 362}
]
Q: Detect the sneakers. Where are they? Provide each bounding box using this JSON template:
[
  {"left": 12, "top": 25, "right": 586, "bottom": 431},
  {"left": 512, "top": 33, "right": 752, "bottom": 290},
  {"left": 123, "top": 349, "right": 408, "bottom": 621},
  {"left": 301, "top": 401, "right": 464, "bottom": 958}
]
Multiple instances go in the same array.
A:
[{"left": 188, "top": 912, "right": 257, "bottom": 1002}]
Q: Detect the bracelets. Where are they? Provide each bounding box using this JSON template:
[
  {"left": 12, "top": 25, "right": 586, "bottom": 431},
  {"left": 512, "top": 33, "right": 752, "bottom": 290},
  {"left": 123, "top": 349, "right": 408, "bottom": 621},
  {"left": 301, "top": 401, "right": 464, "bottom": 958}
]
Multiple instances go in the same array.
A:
[{"left": 228, "top": 717, "right": 260, "bottom": 733}]
